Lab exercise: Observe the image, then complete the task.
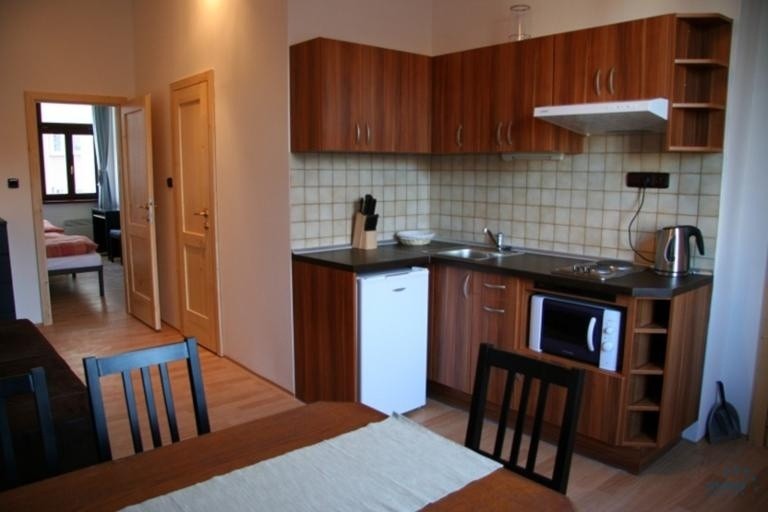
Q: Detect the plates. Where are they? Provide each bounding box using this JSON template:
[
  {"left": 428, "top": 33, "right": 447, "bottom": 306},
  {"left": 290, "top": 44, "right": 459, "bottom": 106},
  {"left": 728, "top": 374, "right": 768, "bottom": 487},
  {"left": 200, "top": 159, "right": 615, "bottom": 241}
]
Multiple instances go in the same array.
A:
[{"left": 396, "top": 228, "right": 436, "bottom": 246}]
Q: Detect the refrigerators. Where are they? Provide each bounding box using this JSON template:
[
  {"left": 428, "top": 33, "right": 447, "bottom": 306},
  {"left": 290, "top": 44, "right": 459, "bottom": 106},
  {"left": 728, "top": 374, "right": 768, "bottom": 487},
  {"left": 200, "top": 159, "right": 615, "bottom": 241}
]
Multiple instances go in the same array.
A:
[{"left": 355, "top": 266, "right": 429, "bottom": 416}]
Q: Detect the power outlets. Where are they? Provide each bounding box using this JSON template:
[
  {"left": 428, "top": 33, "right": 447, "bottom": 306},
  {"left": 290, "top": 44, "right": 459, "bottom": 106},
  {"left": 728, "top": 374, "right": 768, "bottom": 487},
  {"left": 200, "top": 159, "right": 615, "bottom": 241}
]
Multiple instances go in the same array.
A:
[{"left": 626, "top": 173, "right": 670, "bottom": 187}]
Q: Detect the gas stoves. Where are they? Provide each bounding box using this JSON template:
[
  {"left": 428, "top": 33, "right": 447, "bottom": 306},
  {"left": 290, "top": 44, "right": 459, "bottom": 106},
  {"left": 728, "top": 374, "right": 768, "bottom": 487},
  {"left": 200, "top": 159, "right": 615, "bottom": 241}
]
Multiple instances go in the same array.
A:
[{"left": 551, "top": 259, "right": 650, "bottom": 285}]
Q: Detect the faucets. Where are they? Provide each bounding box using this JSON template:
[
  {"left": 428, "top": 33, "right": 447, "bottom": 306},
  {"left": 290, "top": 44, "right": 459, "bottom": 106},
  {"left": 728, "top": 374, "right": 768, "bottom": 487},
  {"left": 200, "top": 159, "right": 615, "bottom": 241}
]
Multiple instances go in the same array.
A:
[{"left": 481, "top": 224, "right": 498, "bottom": 249}]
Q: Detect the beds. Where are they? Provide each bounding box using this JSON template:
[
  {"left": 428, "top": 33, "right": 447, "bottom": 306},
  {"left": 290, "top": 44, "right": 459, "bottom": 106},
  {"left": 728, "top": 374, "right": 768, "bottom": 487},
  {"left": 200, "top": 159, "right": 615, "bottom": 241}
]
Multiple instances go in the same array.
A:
[{"left": 42, "top": 218, "right": 103, "bottom": 296}]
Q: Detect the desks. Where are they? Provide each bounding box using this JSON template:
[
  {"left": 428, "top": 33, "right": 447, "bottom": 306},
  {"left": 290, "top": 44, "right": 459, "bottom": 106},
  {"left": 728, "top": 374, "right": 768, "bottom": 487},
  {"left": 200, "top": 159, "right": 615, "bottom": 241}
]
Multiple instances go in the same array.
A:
[{"left": 0, "top": 397, "right": 578, "bottom": 511}]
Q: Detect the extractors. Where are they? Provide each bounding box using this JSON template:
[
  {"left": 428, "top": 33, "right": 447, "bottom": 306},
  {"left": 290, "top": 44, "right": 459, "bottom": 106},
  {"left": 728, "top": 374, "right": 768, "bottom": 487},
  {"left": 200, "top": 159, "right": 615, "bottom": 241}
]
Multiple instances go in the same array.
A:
[{"left": 533, "top": 97, "right": 668, "bottom": 139}]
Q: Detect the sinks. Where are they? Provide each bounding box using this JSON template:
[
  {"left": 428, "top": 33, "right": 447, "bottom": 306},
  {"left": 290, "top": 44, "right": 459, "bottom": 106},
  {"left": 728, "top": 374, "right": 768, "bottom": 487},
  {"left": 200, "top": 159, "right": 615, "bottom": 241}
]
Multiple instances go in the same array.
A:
[{"left": 439, "top": 247, "right": 492, "bottom": 262}]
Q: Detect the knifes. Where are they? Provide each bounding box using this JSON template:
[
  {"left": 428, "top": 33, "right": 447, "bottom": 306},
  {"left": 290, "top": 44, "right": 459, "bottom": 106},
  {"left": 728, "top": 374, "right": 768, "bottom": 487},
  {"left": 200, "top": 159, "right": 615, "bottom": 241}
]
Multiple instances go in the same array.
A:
[{"left": 358, "top": 194, "right": 378, "bottom": 231}]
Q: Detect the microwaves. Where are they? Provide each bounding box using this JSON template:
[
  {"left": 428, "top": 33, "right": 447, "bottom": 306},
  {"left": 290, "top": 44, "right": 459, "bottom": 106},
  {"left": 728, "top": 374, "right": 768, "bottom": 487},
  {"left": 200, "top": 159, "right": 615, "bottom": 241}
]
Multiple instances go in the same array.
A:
[{"left": 528, "top": 294, "right": 626, "bottom": 372}]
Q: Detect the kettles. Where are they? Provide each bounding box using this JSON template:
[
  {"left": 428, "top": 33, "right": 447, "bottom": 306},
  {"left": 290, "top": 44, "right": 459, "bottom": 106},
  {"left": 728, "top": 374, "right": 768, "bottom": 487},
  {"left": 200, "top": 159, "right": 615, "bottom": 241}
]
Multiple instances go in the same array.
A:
[{"left": 653, "top": 225, "right": 707, "bottom": 278}]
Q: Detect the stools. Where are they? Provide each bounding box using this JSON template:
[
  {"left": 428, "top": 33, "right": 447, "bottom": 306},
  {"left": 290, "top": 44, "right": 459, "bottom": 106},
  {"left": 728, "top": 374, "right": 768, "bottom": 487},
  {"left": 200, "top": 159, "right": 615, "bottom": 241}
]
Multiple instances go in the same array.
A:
[{"left": 110, "top": 229, "right": 123, "bottom": 265}]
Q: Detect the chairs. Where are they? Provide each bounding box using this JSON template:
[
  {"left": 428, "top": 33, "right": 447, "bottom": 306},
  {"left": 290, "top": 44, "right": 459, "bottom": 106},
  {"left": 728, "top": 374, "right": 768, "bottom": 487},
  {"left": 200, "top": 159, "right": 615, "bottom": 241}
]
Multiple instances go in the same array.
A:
[
  {"left": 82, "top": 336, "right": 211, "bottom": 461},
  {"left": 463, "top": 342, "right": 586, "bottom": 496},
  {"left": 1, "top": 366, "right": 63, "bottom": 489}
]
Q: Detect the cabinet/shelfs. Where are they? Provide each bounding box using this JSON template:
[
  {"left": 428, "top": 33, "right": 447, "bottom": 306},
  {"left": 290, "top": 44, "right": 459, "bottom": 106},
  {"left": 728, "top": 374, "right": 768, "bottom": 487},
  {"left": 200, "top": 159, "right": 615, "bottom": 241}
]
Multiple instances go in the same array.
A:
[
  {"left": 514, "top": 273, "right": 634, "bottom": 470},
  {"left": 464, "top": 35, "right": 583, "bottom": 154},
  {"left": 92, "top": 207, "right": 120, "bottom": 258},
  {"left": 432, "top": 52, "right": 464, "bottom": 154},
  {"left": 0, "top": 218, "right": 16, "bottom": 322},
  {"left": 401, "top": 50, "right": 432, "bottom": 153},
  {"left": 427, "top": 263, "right": 519, "bottom": 436},
  {"left": 620, "top": 283, "right": 713, "bottom": 477},
  {"left": 288, "top": 37, "right": 401, "bottom": 153},
  {"left": 668, "top": 13, "right": 733, "bottom": 152},
  {"left": 553, "top": 14, "right": 673, "bottom": 106}
]
